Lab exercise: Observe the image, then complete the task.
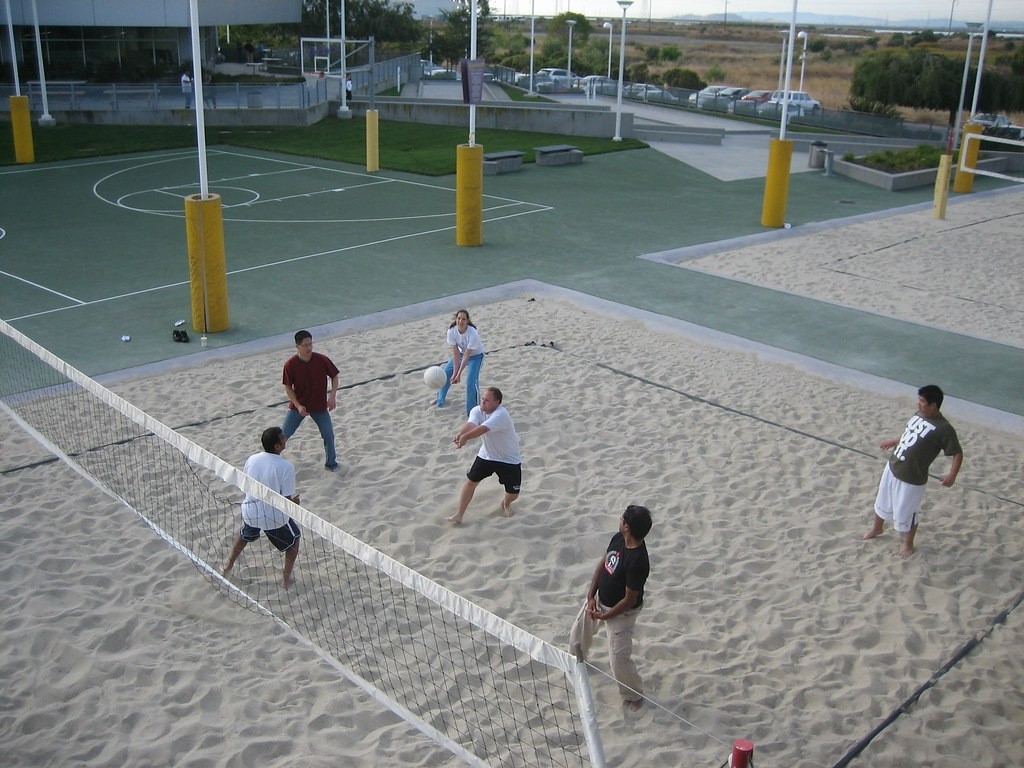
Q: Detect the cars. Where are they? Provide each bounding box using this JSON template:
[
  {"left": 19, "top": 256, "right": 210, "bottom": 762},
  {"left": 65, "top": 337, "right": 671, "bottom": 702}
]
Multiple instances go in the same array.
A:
[
  {"left": 963, "top": 112, "right": 1024, "bottom": 139},
  {"left": 420, "top": 58, "right": 821, "bottom": 124}
]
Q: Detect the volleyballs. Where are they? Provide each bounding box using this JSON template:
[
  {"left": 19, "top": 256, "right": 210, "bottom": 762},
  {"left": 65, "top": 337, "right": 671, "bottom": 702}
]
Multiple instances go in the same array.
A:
[{"left": 423, "top": 366, "right": 448, "bottom": 389}]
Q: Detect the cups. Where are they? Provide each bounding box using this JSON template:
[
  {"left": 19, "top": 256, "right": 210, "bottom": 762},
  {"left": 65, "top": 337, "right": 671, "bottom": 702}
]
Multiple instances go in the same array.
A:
[{"left": 201, "top": 337, "right": 208, "bottom": 347}]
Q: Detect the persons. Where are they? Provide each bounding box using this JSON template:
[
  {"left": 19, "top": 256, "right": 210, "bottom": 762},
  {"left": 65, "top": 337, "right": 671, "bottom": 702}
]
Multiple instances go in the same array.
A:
[
  {"left": 236, "top": 40, "right": 242, "bottom": 51},
  {"left": 346, "top": 73, "right": 352, "bottom": 100},
  {"left": 445, "top": 386, "right": 522, "bottom": 523},
  {"left": 281, "top": 330, "right": 340, "bottom": 472},
  {"left": 566, "top": 505, "right": 652, "bottom": 711},
  {"left": 182, "top": 70, "right": 193, "bottom": 108},
  {"left": 860, "top": 384, "right": 963, "bottom": 559},
  {"left": 244, "top": 39, "right": 254, "bottom": 63},
  {"left": 257, "top": 41, "right": 265, "bottom": 58},
  {"left": 203, "top": 69, "right": 216, "bottom": 110},
  {"left": 429, "top": 309, "right": 485, "bottom": 419},
  {"left": 220, "top": 427, "right": 301, "bottom": 588}
]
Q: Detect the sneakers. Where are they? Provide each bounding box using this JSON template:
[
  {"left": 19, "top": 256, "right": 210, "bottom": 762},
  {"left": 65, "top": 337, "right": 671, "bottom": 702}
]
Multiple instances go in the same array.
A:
[{"left": 172, "top": 329, "right": 189, "bottom": 343}]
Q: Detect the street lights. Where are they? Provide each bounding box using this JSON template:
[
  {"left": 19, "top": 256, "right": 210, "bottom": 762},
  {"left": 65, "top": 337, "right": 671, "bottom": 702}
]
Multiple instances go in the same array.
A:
[
  {"left": 427, "top": 15, "right": 435, "bottom": 63},
  {"left": 777, "top": 30, "right": 790, "bottom": 106},
  {"left": 612, "top": 0, "right": 634, "bottom": 141},
  {"left": 952, "top": 21, "right": 985, "bottom": 149},
  {"left": 565, "top": 20, "right": 577, "bottom": 77}
]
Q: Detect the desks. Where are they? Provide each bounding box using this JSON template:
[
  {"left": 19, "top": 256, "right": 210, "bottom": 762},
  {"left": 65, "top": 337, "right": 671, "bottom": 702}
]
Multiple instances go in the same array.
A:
[{"left": 247, "top": 62, "right": 262, "bottom": 76}]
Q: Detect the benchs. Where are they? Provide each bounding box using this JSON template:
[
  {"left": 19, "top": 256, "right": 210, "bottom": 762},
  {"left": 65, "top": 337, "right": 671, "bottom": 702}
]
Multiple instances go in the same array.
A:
[
  {"left": 104, "top": 89, "right": 161, "bottom": 109},
  {"left": 22, "top": 90, "right": 85, "bottom": 108},
  {"left": 485, "top": 151, "right": 528, "bottom": 172},
  {"left": 533, "top": 143, "right": 577, "bottom": 165}
]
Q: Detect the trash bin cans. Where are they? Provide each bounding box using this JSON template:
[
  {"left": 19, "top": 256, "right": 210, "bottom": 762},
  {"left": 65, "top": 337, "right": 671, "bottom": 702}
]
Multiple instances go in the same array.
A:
[{"left": 809, "top": 139, "right": 828, "bottom": 168}]
